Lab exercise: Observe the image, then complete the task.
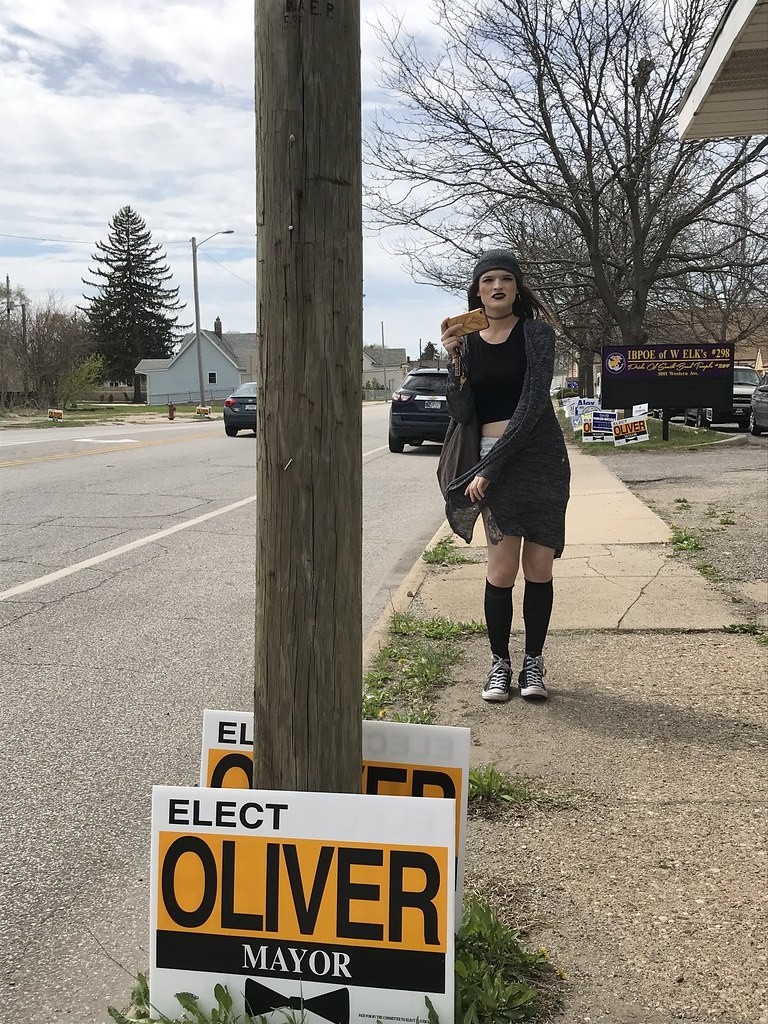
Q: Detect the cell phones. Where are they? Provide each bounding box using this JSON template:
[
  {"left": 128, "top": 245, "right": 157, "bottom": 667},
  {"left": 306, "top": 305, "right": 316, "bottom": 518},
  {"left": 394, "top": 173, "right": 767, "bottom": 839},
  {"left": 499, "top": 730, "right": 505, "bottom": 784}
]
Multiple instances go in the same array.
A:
[{"left": 447, "top": 308, "right": 490, "bottom": 337}]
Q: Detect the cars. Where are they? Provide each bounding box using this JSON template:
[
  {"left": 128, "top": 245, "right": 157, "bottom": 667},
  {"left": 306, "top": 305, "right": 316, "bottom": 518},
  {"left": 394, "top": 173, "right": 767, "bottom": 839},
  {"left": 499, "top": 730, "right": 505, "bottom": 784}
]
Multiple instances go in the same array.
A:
[
  {"left": 749, "top": 371, "right": 768, "bottom": 436},
  {"left": 223, "top": 382, "right": 257, "bottom": 436},
  {"left": 550, "top": 386, "right": 565, "bottom": 394}
]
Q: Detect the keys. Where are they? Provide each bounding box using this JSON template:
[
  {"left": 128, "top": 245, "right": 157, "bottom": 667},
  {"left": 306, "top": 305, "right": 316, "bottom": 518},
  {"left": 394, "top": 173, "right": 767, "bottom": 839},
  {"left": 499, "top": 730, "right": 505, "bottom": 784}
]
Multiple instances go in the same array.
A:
[{"left": 459, "top": 376, "right": 466, "bottom": 390}]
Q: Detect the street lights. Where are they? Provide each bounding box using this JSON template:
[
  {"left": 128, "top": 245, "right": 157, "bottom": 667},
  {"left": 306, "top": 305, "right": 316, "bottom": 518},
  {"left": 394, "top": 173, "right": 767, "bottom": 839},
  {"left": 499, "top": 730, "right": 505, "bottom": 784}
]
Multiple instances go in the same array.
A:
[
  {"left": 419, "top": 338, "right": 437, "bottom": 366},
  {"left": 192, "top": 230, "right": 234, "bottom": 415}
]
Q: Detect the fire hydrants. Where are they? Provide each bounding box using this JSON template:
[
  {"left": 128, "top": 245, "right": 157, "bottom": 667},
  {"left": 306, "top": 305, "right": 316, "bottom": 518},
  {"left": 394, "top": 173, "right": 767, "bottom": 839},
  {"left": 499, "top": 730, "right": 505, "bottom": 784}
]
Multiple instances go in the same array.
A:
[{"left": 168, "top": 402, "right": 176, "bottom": 420}]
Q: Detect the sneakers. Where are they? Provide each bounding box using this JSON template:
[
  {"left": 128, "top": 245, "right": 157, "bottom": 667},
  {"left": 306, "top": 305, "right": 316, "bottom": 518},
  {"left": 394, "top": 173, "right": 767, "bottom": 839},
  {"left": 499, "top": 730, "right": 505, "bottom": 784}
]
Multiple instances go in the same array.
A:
[
  {"left": 481, "top": 657, "right": 512, "bottom": 702},
  {"left": 518, "top": 654, "right": 549, "bottom": 697}
]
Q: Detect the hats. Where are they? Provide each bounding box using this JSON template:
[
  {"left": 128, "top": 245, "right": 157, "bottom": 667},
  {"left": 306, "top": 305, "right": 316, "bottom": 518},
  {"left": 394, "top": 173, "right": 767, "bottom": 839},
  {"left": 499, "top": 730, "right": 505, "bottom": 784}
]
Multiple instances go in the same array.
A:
[{"left": 472, "top": 249, "right": 523, "bottom": 291}]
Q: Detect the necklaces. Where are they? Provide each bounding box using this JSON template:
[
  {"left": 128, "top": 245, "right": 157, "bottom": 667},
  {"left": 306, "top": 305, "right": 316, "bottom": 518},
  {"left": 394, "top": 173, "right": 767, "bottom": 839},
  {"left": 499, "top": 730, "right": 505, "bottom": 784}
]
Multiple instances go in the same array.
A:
[{"left": 486, "top": 312, "right": 513, "bottom": 321}]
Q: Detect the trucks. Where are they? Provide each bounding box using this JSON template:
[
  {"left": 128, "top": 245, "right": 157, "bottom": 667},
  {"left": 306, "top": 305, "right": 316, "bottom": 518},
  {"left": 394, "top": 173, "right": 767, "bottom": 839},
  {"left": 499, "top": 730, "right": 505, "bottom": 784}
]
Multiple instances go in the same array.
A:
[{"left": 594, "top": 372, "right": 601, "bottom": 403}]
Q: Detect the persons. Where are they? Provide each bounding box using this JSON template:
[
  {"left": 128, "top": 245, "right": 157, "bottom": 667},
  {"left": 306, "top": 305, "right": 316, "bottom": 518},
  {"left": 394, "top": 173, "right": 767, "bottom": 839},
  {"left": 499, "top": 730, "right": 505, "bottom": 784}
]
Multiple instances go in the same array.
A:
[{"left": 437, "top": 250, "right": 572, "bottom": 700}]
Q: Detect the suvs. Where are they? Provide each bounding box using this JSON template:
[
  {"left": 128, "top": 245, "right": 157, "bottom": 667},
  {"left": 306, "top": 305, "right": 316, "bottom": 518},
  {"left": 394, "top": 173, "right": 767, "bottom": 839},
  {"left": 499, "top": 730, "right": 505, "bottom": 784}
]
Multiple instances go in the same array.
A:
[
  {"left": 684, "top": 364, "right": 761, "bottom": 427},
  {"left": 389, "top": 367, "right": 452, "bottom": 453}
]
